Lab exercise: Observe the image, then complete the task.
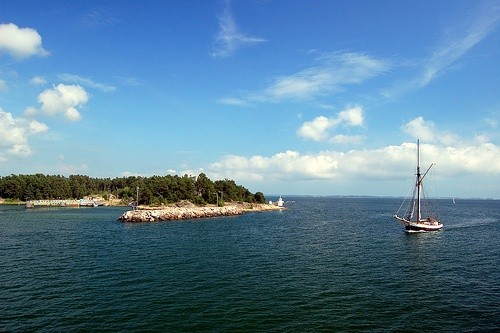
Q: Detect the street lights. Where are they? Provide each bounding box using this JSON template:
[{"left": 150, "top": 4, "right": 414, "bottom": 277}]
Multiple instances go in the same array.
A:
[
  {"left": 213, "top": 193, "right": 218, "bottom": 207},
  {"left": 136, "top": 186, "right": 140, "bottom": 211}
]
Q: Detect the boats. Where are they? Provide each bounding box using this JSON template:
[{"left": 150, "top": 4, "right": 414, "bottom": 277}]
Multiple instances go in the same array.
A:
[
  {"left": 47, "top": 202, "right": 66, "bottom": 207},
  {"left": 78, "top": 199, "right": 103, "bottom": 207},
  {"left": 278, "top": 195, "right": 284, "bottom": 207}
]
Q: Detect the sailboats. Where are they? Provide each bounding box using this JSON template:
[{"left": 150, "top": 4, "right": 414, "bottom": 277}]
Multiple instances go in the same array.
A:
[{"left": 392, "top": 139, "right": 444, "bottom": 234}]
